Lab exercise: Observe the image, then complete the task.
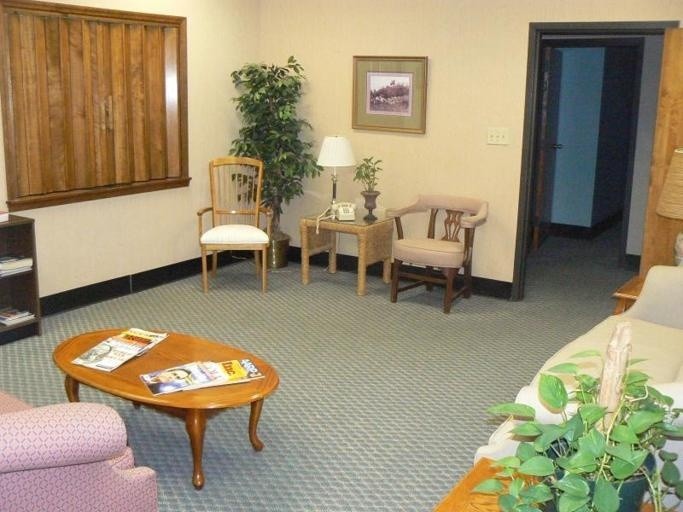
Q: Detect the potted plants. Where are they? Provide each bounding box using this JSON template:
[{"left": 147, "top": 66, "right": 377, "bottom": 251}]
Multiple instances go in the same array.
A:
[
  {"left": 229, "top": 56, "right": 324, "bottom": 269},
  {"left": 468, "top": 348, "right": 683, "bottom": 512},
  {"left": 352, "top": 155, "right": 383, "bottom": 222}
]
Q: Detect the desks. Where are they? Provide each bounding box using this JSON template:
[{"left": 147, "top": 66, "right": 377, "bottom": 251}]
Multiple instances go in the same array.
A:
[
  {"left": 300, "top": 215, "right": 394, "bottom": 296},
  {"left": 436, "top": 456, "right": 667, "bottom": 512}
]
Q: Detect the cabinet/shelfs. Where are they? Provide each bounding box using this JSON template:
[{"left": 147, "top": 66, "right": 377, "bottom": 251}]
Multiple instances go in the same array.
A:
[{"left": 0, "top": 214, "right": 42, "bottom": 346}]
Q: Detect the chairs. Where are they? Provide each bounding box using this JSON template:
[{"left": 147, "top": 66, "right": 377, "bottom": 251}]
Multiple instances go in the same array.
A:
[
  {"left": 385, "top": 194, "right": 489, "bottom": 313},
  {"left": 197, "top": 156, "right": 269, "bottom": 293}
]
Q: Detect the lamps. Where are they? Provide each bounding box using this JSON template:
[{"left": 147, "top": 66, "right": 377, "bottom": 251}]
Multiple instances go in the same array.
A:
[
  {"left": 656, "top": 148, "right": 683, "bottom": 266},
  {"left": 316, "top": 135, "right": 354, "bottom": 204}
]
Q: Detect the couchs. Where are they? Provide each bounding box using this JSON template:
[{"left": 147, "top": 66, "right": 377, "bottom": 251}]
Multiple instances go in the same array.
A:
[
  {"left": 1, "top": 391, "right": 158, "bottom": 512},
  {"left": 475, "top": 264, "right": 683, "bottom": 512}
]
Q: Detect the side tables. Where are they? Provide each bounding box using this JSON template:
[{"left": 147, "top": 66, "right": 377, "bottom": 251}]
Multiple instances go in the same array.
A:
[{"left": 610, "top": 276, "right": 646, "bottom": 314}]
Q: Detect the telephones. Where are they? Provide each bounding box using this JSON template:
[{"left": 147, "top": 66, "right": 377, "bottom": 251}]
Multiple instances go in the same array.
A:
[{"left": 331, "top": 202, "right": 356, "bottom": 223}]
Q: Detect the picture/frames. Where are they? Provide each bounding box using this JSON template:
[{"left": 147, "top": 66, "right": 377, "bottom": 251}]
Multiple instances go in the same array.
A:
[{"left": 351, "top": 55, "right": 428, "bottom": 135}]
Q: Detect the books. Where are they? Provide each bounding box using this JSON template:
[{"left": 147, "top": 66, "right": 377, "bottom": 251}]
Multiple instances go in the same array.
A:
[
  {"left": 0, "top": 255, "right": 33, "bottom": 279},
  {"left": 71, "top": 327, "right": 169, "bottom": 372},
  {"left": 0, "top": 306, "right": 35, "bottom": 327},
  {"left": 0, "top": 210, "right": 9, "bottom": 223},
  {"left": 139, "top": 357, "right": 266, "bottom": 397}
]
